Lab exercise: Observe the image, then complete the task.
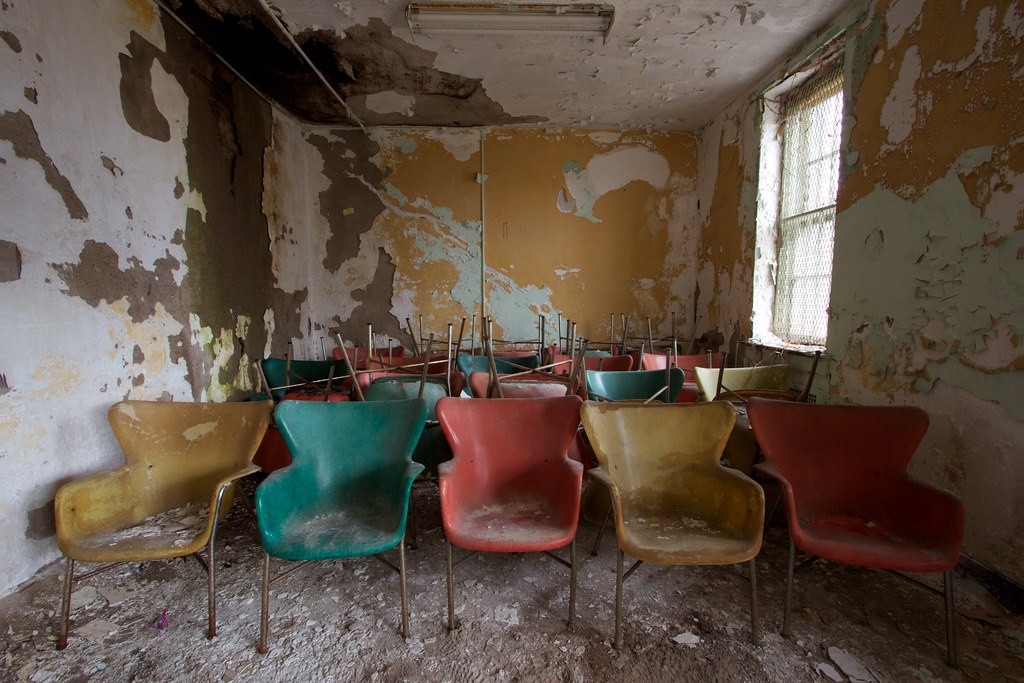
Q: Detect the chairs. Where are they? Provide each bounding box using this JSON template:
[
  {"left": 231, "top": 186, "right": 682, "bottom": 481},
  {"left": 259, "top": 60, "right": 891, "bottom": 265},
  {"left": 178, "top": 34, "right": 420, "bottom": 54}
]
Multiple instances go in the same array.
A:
[
  {"left": 746, "top": 397, "right": 966, "bottom": 670},
  {"left": 247, "top": 314, "right": 822, "bottom": 558},
  {"left": 435, "top": 395, "right": 584, "bottom": 631},
  {"left": 56, "top": 399, "right": 274, "bottom": 645},
  {"left": 579, "top": 399, "right": 766, "bottom": 651},
  {"left": 255, "top": 397, "right": 429, "bottom": 655}
]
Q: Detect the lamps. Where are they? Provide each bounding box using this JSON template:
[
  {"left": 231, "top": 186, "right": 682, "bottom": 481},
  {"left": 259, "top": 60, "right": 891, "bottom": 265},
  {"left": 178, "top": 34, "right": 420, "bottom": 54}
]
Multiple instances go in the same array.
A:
[{"left": 405, "top": 2, "right": 616, "bottom": 46}]
